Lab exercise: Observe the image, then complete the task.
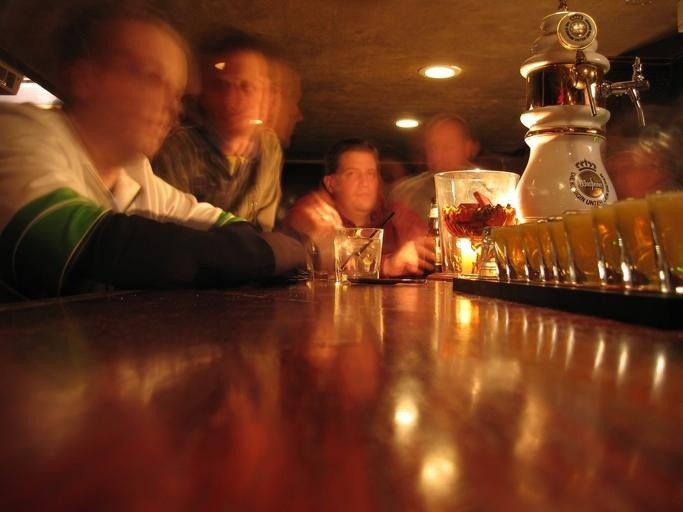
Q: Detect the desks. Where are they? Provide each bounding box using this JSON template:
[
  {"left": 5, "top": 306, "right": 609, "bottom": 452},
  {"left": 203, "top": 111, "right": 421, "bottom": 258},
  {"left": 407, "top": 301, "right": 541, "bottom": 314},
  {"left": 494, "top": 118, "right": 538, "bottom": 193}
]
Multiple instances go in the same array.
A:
[{"left": 0, "top": 281, "right": 681, "bottom": 512}]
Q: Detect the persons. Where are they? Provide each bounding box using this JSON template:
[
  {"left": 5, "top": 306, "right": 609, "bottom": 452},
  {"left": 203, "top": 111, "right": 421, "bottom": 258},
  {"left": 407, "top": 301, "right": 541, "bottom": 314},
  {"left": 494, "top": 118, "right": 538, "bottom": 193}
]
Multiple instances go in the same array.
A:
[{"left": 0, "top": 0, "right": 682, "bottom": 298}]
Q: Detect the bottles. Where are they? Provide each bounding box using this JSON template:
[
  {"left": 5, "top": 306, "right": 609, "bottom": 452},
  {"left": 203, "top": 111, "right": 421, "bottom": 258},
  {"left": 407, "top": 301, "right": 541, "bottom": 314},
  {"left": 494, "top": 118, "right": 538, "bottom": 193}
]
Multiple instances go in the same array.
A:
[{"left": 424, "top": 196, "right": 443, "bottom": 273}]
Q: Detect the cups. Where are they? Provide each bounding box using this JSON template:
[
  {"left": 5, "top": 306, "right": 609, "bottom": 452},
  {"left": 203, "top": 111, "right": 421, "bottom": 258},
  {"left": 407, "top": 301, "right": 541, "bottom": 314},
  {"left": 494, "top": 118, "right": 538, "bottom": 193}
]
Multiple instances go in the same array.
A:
[
  {"left": 491, "top": 186, "right": 683, "bottom": 293},
  {"left": 334, "top": 228, "right": 384, "bottom": 283},
  {"left": 434, "top": 169, "right": 522, "bottom": 280}
]
